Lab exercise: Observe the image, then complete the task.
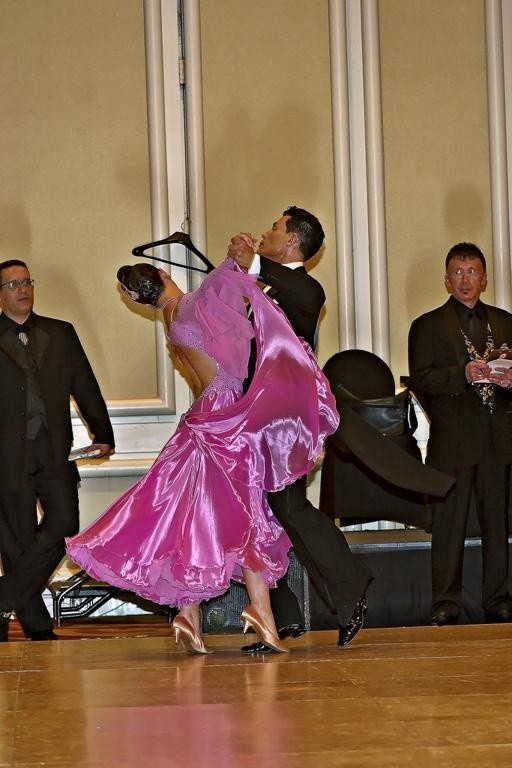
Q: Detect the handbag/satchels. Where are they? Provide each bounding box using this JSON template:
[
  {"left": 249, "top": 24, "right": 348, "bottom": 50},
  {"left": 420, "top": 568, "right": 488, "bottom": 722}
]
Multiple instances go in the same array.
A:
[{"left": 332, "top": 383, "right": 418, "bottom": 455}]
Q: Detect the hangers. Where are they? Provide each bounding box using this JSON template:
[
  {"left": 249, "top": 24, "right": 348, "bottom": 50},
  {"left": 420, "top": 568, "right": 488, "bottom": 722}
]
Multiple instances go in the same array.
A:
[{"left": 131, "top": 217, "right": 216, "bottom": 273}]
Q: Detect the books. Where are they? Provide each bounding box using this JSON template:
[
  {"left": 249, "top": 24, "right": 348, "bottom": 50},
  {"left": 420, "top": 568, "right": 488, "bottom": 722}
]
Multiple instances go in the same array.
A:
[{"left": 68, "top": 446, "right": 101, "bottom": 462}]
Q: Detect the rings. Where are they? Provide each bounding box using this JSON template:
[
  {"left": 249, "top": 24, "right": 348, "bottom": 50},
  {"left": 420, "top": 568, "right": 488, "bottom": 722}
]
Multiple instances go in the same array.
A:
[
  {"left": 500, "top": 380, "right": 504, "bottom": 385},
  {"left": 477, "top": 375, "right": 482, "bottom": 377}
]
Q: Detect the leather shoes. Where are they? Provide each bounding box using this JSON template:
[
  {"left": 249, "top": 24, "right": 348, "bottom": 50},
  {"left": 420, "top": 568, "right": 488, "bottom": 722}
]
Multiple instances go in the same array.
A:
[
  {"left": 338, "top": 596, "right": 369, "bottom": 648},
  {"left": 31, "top": 630, "right": 61, "bottom": 640},
  {"left": 240, "top": 604, "right": 291, "bottom": 652},
  {"left": 241, "top": 623, "right": 307, "bottom": 654},
  {"left": 432, "top": 612, "right": 455, "bottom": 626},
  {"left": 173, "top": 615, "right": 214, "bottom": 656},
  {"left": 494, "top": 610, "right": 512, "bottom": 622},
  {"left": 0, "top": 610, "right": 9, "bottom": 641}
]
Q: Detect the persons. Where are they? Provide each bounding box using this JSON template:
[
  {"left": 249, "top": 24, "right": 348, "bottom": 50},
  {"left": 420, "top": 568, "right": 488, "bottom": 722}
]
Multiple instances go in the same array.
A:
[
  {"left": 65, "top": 231, "right": 342, "bottom": 656},
  {"left": 406, "top": 241, "right": 511, "bottom": 628},
  {"left": 1, "top": 259, "right": 119, "bottom": 643},
  {"left": 227, "top": 204, "right": 458, "bottom": 656}
]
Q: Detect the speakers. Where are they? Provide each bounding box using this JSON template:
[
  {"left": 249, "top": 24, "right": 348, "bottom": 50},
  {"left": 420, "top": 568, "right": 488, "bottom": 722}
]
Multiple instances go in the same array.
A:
[{"left": 202, "top": 545, "right": 305, "bottom": 635}]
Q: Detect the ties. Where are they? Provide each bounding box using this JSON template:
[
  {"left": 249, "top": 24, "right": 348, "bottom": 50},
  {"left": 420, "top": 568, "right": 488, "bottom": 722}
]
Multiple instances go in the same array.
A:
[
  {"left": 469, "top": 310, "right": 483, "bottom": 359},
  {"left": 17, "top": 326, "right": 29, "bottom": 347}
]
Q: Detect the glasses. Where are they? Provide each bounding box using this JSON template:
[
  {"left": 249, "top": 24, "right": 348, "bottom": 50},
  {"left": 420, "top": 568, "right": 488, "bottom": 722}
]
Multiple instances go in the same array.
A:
[{"left": 1, "top": 278, "right": 36, "bottom": 290}]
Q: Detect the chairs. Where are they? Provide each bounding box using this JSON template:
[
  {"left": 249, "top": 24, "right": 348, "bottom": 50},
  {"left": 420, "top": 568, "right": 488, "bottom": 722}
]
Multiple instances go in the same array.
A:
[{"left": 318, "top": 348, "right": 434, "bottom": 535}]
